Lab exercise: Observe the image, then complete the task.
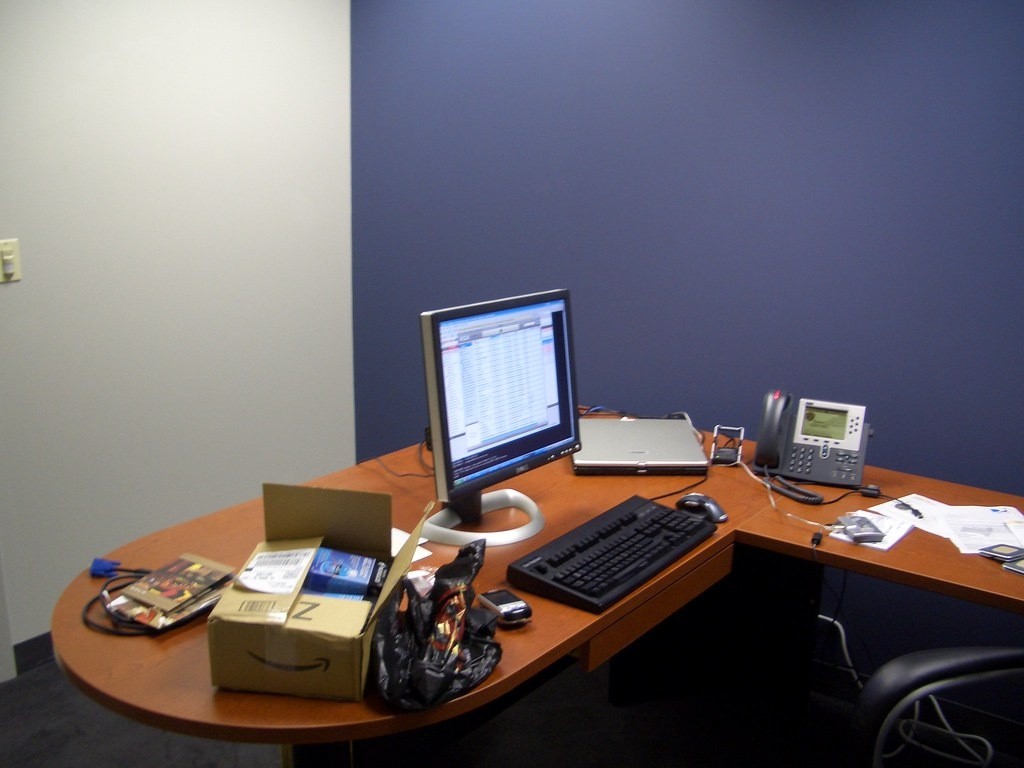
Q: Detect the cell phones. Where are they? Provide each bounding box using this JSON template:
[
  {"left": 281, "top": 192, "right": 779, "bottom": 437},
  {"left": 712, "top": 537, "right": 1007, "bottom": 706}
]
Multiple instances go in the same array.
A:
[{"left": 478, "top": 589, "right": 532, "bottom": 627}]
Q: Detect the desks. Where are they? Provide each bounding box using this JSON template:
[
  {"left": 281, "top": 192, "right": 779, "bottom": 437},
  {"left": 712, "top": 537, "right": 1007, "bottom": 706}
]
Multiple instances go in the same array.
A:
[{"left": 53, "top": 405, "right": 1024, "bottom": 768}]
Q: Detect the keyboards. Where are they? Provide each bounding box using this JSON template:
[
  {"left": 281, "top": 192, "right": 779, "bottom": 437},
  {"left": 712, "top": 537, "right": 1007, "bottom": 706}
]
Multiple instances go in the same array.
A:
[{"left": 507, "top": 495, "right": 718, "bottom": 614}]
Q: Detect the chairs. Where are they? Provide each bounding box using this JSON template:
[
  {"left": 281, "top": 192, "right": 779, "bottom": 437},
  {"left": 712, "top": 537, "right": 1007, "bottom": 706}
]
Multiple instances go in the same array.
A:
[{"left": 848, "top": 645, "right": 1024, "bottom": 768}]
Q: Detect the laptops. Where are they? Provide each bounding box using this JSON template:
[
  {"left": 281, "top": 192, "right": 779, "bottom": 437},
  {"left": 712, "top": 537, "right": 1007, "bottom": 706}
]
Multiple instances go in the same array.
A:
[{"left": 572, "top": 419, "right": 709, "bottom": 476}]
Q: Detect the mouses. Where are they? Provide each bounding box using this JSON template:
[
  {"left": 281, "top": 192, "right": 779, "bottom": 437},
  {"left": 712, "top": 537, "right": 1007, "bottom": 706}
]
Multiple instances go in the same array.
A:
[{"left": 675, "top": 493, "right": 727, "bottom": 524}]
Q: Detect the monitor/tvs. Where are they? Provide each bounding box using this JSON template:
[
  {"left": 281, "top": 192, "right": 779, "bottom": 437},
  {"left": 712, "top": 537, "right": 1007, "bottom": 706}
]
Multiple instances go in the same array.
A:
[{"left": 420, "top": 288, "right": 582, "bottom": 547}]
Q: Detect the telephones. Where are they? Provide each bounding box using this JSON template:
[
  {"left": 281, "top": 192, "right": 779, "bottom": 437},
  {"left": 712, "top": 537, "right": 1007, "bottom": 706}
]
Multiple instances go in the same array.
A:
[{"left": 750, "top": 387, "right": 875, "bottom": 487}]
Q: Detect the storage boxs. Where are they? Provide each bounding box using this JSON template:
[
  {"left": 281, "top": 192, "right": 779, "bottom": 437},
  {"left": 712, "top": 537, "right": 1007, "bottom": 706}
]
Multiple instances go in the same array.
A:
[{"left": 207, "top": 483, "right": 435, "bottom": 702}]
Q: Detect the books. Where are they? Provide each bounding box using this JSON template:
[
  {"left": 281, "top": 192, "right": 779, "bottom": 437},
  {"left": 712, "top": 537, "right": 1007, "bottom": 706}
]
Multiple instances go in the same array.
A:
[{"left": 106, "top": 551, "right": 237, "bottom": 631}]
